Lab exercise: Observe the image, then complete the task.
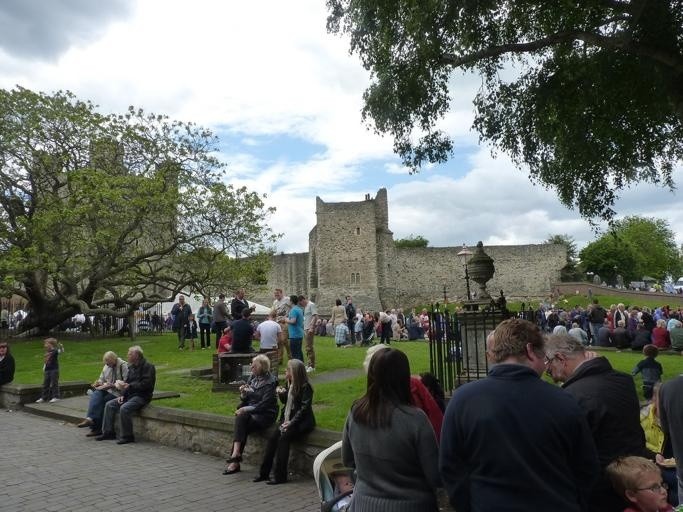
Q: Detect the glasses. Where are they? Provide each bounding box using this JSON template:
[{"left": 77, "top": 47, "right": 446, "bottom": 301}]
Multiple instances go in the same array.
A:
[
  {"left": 637, "top": 483, "right": 669, "bottom": 492},
  {"left": 541, "top": 352, "right": 551, "bottom": 367}
]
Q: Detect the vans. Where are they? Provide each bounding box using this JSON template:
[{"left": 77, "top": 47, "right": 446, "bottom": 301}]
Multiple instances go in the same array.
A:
[{"left": 673, "top": 277, "right": 682, "bottom": 294}]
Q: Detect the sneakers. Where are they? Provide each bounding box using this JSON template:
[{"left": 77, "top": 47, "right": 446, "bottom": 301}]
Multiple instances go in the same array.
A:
[
  {"left": 36, "top": 398, "right": 45, "bottom": 403},
  {"left": 305, "top": 366, "right": 315, "bottom": 373},
  {"left": 49, "top": 398, "right": 60, "bottom": 403},
  {"left": 78, "top": 419, "right": 95, "bottom": 427}
]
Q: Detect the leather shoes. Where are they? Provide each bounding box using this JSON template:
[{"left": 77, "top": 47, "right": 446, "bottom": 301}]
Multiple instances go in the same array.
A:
[
  {"left": 265, "top": 478, "right": 276, "bottom": 484},
  {"left": 86, "top": 429, "right": 134, "bottom": 444},
  {"left": 226, "top": 455, "right": 243, "bottom": 463},
  {"left": 222, "top": 465, "right": 240, "bottom": 474},
  {"left": 253, "top": 476, "right": 260, "bottom": 481}
]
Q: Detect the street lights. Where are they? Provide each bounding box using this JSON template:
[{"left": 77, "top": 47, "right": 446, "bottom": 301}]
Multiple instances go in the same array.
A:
[{"left": 455, "top": 242, "right": 473, "bottom": 301}]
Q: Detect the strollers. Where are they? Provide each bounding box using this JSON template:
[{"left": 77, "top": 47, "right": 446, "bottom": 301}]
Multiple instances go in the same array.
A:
[{"left": 311, "top": 433, "right": 358, "bottom": 512}]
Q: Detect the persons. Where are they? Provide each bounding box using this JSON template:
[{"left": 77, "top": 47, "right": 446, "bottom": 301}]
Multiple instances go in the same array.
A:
[
  {"left": 34, "top": 338, "right": 61, "bottom": 402},
  {"left": 75, "top": 348, "right": 128, "bottom": 436},
  {"left": 656, "top": 372, "right": 682, "bottom": 500},
  {"left": 436, "top": 317, "right": 604, "bottom": 510},
  {"left": 315, "top": 295, "right": 462, "bottom": 348},
  {"left": 0, "top": 342, "right": 15, "bottom": 386},
  {"left": 520, "top": 281, "right": 682, "bottom": 353},
  {"left": 0, "top": 293, "right": 161, "bottom": 333},
  {"left": 333, "top": 475, "right": 354, "bottom": 510},
  {"left": 341, "top": 348, "right": 445, "bottom": 511},
  {"left": 417, "top": 371, "right": 447, "bottom": 415},
  {"left": 364, "top": 341, "right": 446, "bottom": 445},
  {"left": 222, "top": 354, "right": 281, "bottom": 473},
  {"left": 161, "top": 286, "right": 318, "bottom": 375},
  {"left": 638, "top": 382, "right": 673, "bottom": 465},
  {"left": 629, "top": 345, "right": 663, "bottom": 397},
  {"left": 545, "top": 327, "right": 645, "bottom": 461},
  {"left": 607, "top": 455, "right": 677, "bottom": 511},
  {"left": 251, "top": 357, "right": 315, "bottom": 484},
  {"left": 97, "top": 344, "right": 157, "bottom": 445}
]
